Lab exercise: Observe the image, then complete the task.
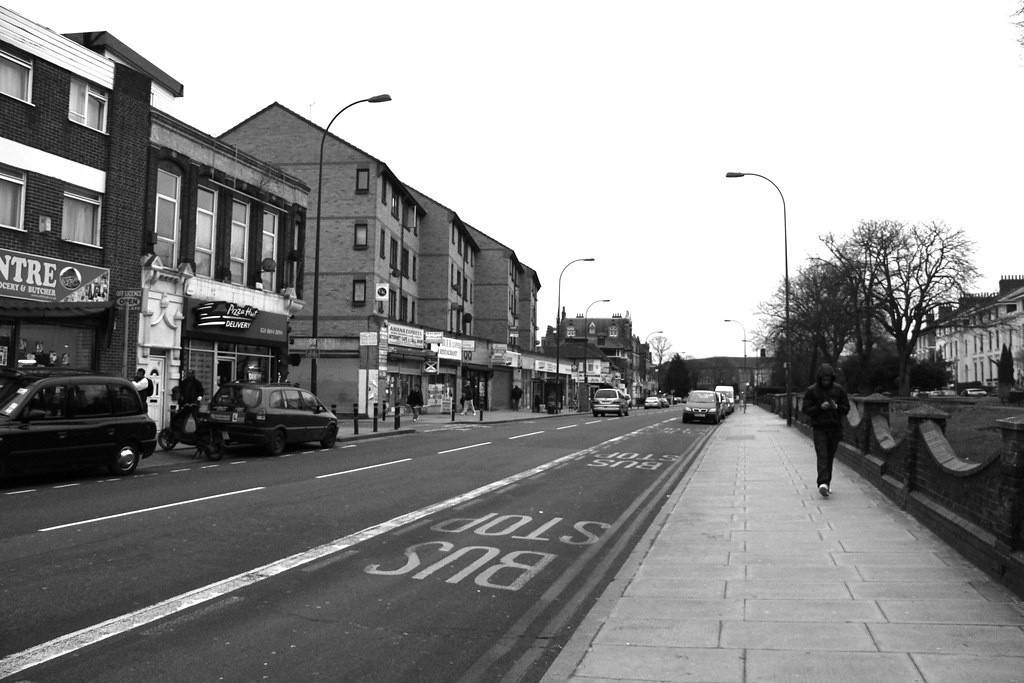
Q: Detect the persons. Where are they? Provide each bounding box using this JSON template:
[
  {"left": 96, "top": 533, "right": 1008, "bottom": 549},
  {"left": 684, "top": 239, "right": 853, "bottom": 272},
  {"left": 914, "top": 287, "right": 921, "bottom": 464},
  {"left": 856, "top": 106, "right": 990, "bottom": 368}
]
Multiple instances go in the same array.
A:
[
  {"left": 803, "top": 364, "right": 851, "bottom": 497},
  {"left": 131, "top": 368, "right": 149, "bottom": 414},
  {"left": 407, "top": 385, "right": 423, "bottom": 422},
  {"left": 179, "top": 368, "right": 204, "bottom": 423},
  {"left": 461, "top": 385, "right": 476, "bottom": 415},
  {"left": 512, "top": 385, "right": 523, "bottom": 411}
]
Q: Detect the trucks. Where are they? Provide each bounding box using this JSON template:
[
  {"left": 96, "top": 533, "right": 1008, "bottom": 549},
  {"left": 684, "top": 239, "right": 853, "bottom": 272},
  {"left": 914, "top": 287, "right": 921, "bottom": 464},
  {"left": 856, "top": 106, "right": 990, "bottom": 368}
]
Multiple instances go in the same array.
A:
[{"left": 714, "top": 385, "right": 735, "bottom": 413}]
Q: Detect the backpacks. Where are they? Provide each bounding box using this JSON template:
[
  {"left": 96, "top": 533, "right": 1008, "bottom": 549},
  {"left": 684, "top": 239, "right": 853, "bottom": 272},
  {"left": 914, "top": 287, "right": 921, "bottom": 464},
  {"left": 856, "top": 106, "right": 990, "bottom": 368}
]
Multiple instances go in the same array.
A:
[{"left": 139, "top": 377, "right": 154, "bottom": 396}]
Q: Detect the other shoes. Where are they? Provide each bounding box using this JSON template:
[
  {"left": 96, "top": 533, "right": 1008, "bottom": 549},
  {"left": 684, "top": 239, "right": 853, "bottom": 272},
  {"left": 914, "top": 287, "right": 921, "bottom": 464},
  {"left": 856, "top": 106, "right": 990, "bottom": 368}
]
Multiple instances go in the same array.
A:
[
  {"left": 461, "top": 411, "right": 467, "bottom": 415},
  {"left": 473, "top": 411, "right": 476, "bottom": 415},
  {"left": 413, "top": 416, "right": 419, "bottom": 422},
  {"left": 819, "top": 484, "right": 830, "bottom": 496}
]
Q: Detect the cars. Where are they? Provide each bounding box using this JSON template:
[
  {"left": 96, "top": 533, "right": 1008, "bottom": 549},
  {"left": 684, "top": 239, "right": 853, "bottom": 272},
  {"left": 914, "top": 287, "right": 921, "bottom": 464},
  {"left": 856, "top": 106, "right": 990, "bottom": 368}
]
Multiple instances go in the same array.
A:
[
  {"left": 205, "top": 377, "right": 341, "bottom": 459},
  {"left": 643, "top": 393, "right": 687, "bottom": 410},
  {"left": 960, "top": 387, "right": 988, "bottom": 397},
  {"left": 714, "top": 391, "right": 733, "bottom": 420},
  {"left": 681, "top": 389, "right": 721, "bottom": 424},
  {"left": 0, "top": 362, "right": 159, "bottom": 489},
  {"left": 589, "top": 387, "right": 630, "bottom": 417}
]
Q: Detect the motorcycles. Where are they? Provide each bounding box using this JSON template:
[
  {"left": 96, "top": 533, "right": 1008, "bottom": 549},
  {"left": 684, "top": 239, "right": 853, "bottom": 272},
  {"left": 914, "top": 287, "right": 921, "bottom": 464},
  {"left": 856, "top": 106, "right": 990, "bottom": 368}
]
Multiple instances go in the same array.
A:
[{"left": 156, "top": 394, "right": 226, "bottom": 463}]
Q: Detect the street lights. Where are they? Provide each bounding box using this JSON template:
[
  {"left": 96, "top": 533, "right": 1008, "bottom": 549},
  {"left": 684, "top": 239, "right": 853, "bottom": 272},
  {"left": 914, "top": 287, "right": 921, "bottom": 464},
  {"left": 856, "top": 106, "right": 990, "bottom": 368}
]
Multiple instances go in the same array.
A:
[
  {"left": 724, "top": 319, "right": 747, "bottom": 411},
  {"left": 642, "top": 330, "right": 663, "bottom": 403},
  {"left": 584, "top": 298, "right": 610, "bottom": 384},
  {"left": 724, "top": 171, "right": 794, "bottom": 430},
  {"left": 310, "top": 92, "right": 394, "bottom": 400},
  {"left": 742, "top": 338, "right": 759, "bottom": 407},
  {"left": 554, "top": 257, "right": 596, "bottom": 415}
]
